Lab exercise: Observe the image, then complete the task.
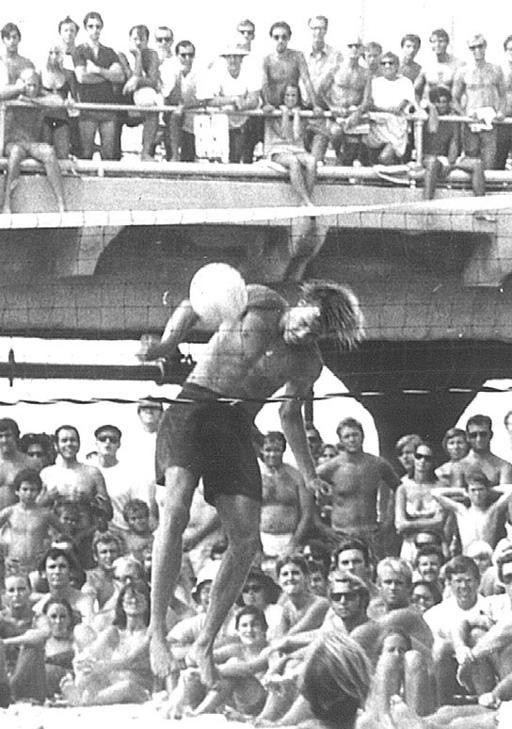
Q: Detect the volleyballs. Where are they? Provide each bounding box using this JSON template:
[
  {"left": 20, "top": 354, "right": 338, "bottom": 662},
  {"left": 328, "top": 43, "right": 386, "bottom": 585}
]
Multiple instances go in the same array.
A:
[{"left": 190, "top": 260, "right": 248, "bottom": 322}]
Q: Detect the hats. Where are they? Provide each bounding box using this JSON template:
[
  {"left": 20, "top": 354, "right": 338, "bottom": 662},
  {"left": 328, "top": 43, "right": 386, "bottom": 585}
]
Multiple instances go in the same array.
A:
[
  {"left": 220, "top": 32, "right": 251, "bottom": 56},
  {"left": 190, "top": 559, "right": 222, "bottom": 604}
]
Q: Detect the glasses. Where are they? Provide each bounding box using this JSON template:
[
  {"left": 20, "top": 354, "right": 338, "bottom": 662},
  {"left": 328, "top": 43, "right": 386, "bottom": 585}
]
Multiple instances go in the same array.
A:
[
  {"left": 238, "top": 29, "right": 254, "bottom": 35},
  {"left": 155, "top": 36, "right": 174, "bottom": 43},
  {"left": 380, "top": 61, "right": 394, "bottom": 66},
  {"left": 414, "top": 452, "right": 435, "bottom": 465},
  {"left": 330, "top": 592, "right": 360, "bottom": 603},
  {"left": 179, "top": 51, "right": 195, "bottom": 58},
  {"left": 271, "top": 34, "right": 289, "bottom": 41},
  {"left": 346, "top": 44, "right": 363, "bottom": 49},
  {"left": 98, "top": 435, "right": 120, "bottom": 444},
  {"left": 87, "top": 23, "right": 102, "bottom": 29}
]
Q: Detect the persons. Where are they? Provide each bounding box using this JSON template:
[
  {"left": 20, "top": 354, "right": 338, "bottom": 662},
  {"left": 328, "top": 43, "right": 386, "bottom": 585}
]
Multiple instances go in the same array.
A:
[
  {"left": 0, "top": 12, "right": 512, "bottom": 213},
  {"left": 0, "top": 389, "right": 512, "bottom": 729},
  {"left": 134, "top": 281, "right": 365, "bottom": 691}
]
